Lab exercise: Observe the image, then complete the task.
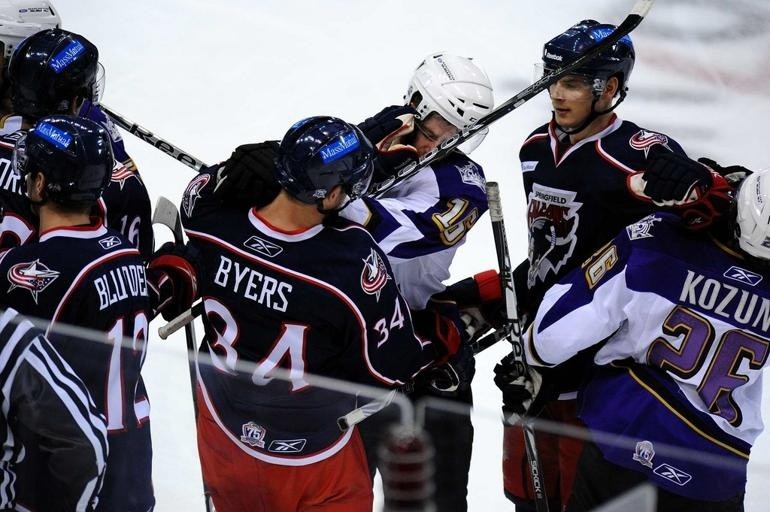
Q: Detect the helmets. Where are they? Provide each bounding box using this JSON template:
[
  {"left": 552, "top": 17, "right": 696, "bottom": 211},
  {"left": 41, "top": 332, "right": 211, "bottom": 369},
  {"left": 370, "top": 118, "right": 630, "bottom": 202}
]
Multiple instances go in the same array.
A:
[
  {"left": 734, "top": 169, "right": 770, "bottom": 260},
  {"left": 8, "top": 28, "right": 113, "bottom": 201},
  {"left": 406, "top": 54, "right": 495, "bottom": 132},
  {"left": 277, "top": 116, "right": 373, "bottom": 203},
  {"left": 543, "top": 21, "right": 634, "bottom": 95}
]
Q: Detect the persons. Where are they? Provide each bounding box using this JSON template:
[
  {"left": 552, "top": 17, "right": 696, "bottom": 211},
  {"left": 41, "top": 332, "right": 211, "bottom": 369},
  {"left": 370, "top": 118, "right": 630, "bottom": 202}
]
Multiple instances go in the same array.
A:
[
  {"left": 0, "top": 27, "right": 153, "bottom": 262},
  {"left": 424, "top": 19, "right": 736, "bottom": 512},
  {"left": 153, "top": 51, "right": 493, "bottom": 512},
  {"left": 494, "top": 157, "right": 770, "bottom": 512},
  {"left": 180, "top": 114, "right": 440, "bottom": 512},
  {"left": 0, "top": 0, "right": 62, "bottom": 114},
  {"left": 0, "top": 113, "right": 159, "bottom": 512}
]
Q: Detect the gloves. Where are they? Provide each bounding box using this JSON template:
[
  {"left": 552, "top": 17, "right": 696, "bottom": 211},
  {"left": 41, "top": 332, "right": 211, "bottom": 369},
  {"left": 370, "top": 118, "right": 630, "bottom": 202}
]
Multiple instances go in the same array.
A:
[
  {"left": 494, "top": 356, "right": 561, "bottom": 426},
  {"left": 220, "top": 141, "right": 280, "bottom": 212},
  {"left": 643, "top": 145, "right": 714, "bottom": 207},
  {"left": 357, "top": 106, "right": 417, "bottom": 181}
]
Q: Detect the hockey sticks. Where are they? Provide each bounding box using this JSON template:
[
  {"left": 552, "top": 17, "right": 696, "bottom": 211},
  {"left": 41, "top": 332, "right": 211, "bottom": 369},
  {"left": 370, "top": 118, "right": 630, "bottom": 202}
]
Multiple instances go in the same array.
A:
[
  {"left": 151, "top": 197, "right": 215, "bottom": 511},
  {"left": 158, "top": 1, "right": 652, "bottom": 340}
]
